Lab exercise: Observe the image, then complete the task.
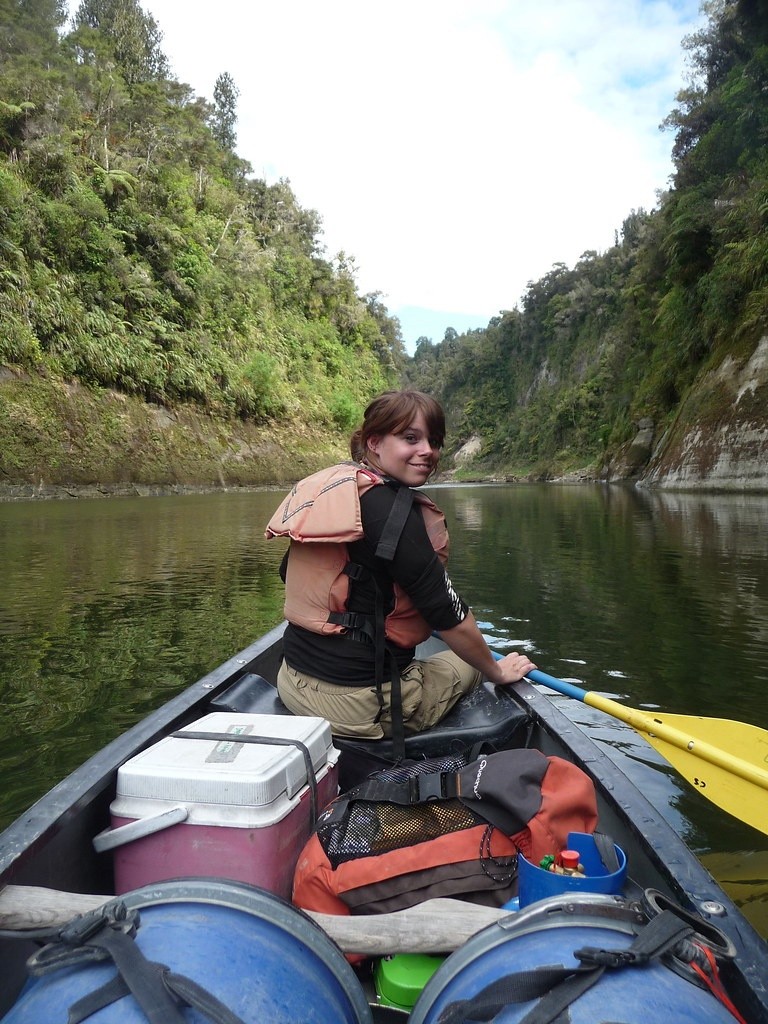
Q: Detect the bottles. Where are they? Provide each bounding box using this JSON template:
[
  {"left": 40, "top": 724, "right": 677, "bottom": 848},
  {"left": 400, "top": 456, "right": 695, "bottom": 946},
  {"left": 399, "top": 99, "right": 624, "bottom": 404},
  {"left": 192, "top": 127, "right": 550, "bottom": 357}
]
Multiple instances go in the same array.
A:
[{"left": 561, "top": 850, "right": 580, "bottom": 876}]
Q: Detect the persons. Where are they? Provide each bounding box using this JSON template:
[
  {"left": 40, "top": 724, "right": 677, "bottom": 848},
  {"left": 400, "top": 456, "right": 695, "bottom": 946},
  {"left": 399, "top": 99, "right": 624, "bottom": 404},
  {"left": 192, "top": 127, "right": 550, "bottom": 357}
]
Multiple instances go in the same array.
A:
[{"left": 264, "top": 390, "right": 538, "bottom": 739}]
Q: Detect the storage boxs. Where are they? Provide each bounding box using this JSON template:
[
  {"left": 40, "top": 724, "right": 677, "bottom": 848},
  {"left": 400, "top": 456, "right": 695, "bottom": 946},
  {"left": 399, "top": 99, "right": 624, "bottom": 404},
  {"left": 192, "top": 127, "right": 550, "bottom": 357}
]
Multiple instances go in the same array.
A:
[
  {"left": 91, "top": 711, "right": 341, "bottom": 903},
  {"left": 371, "top": 952, "right": 446, "bottom": 1013}
]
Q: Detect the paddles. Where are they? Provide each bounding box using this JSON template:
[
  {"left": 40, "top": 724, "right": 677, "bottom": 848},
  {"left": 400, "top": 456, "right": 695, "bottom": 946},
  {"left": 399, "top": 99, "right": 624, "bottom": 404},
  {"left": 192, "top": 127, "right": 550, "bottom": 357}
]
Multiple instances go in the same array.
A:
[{"left": 430, "top": 630, "right": 768, "bottom": 837}]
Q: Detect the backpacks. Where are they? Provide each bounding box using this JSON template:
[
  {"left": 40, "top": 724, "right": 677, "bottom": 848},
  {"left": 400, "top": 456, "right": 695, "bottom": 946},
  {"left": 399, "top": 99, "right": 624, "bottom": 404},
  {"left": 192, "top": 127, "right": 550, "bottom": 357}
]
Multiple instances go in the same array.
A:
[{"left": 293, "top": 748, "right": 599, "bottom": 970}]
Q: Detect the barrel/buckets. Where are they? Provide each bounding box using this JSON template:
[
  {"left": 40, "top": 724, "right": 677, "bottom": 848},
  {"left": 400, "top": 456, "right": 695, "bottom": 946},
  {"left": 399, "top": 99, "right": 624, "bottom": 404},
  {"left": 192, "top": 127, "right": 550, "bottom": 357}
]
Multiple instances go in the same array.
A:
[{"left": 518, "top": 832, "right": 626, "bottom": 912}]
